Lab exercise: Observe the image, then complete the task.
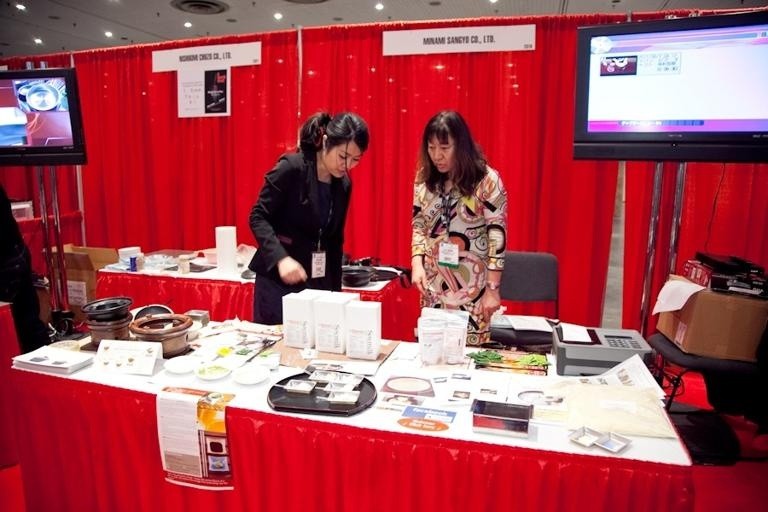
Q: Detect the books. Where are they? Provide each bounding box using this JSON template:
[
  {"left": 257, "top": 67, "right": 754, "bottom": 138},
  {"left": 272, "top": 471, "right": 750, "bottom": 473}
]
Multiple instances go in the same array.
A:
[{"left": 11, "top": 343, "right": 98, "bottom": 378}]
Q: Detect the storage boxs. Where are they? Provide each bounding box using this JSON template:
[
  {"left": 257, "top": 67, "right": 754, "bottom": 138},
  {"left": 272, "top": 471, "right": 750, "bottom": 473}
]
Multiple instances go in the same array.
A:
[{"left": 656, "top": 274, "right": 767, "bottom": 363}]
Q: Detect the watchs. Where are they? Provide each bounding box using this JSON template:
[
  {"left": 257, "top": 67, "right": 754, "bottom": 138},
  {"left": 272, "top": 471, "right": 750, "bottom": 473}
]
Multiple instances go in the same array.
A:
[{"left": 483, "top": 280, "right": 501, "bottom": 290}]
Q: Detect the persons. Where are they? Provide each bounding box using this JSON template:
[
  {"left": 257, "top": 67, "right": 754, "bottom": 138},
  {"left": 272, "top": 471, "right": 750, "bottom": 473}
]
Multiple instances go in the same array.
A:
[
  {"left": 250, "top": 112, "right": 368, "bottom": 328},
  {"left": 1, "top": 183, "right": 50, "bottom": 351},
  {"left": 411, "top": 112, "right": 508, "bottom": 353}
]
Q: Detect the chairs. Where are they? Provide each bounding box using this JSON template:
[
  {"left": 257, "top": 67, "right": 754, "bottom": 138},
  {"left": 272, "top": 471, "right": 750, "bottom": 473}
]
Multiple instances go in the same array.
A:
[{"left": 490, "top": 250, "right": 561, "bottom": 353}]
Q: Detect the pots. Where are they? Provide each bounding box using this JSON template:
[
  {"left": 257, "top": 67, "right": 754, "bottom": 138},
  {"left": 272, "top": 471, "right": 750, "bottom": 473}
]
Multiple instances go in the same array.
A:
[
  {"left": 128, "top": 315, "right": 194, "bottom": 360},
  {"left": 88, "top": 311, "right": 134, "bottom": 347}
]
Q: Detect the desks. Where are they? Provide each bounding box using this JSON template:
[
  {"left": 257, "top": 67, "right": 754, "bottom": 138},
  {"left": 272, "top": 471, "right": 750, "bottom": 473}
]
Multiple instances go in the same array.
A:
[
  {"left": 0, "top": 314, "right": 696, "bottom": 511},
  {"left": 95, "top": 248, "right": 421, "bottom": 345}
]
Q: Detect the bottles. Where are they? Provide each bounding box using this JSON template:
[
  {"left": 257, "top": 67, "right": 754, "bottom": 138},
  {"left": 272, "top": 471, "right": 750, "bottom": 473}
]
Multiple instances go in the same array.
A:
[
  {"left": 177, "top": 255, "right": 191, "bottom": 273},
  {"left": 136, "top": 253, "right": 145, "bottom": 271}
]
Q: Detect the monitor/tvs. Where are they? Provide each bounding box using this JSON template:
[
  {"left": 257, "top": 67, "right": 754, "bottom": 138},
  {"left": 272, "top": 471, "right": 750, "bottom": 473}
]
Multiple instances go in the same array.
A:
[
  {"left": 573, "top": 10, "right": 768, "bottom": 161},
  {"left": 0, "top": 67, "right": 86, "bottom": 165}
]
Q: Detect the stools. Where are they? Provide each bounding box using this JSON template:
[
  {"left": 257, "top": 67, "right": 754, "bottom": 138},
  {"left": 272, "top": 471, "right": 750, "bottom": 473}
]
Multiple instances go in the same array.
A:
[{"left": 648, "top": 335, "right": 742, "bottom": 411}]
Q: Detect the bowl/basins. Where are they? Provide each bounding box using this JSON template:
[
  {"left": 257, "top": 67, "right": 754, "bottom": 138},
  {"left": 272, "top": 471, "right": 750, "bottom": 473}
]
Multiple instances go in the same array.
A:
[
  {"left": 341, "top": 265, "right": 377, "bottom": 286},
  {"left": 81, "top": 297, "right": 132, "bottom": 318},
  {"left": 352, "top": 256, "right": 372, "bottom": 267}
]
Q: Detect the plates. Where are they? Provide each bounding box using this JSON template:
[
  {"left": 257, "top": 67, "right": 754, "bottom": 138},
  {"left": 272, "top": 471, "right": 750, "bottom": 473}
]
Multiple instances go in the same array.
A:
[
  {"left": 233, "top": 365, "right": 270, "bottom": 385},
  {"left": 197, "top": 364, "right": 232, "bottom": 380},
  {"left": 164, "top": 356, "right": 198, "bottom": 372},
  {"left": 370, "top": 269, "right": 398, "bottom": 282}
]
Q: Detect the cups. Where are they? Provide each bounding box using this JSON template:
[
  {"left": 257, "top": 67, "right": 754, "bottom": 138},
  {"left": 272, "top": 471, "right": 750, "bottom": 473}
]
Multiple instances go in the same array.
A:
[{"left": 13, "top": 79, "right": 60, "bottom": 113}]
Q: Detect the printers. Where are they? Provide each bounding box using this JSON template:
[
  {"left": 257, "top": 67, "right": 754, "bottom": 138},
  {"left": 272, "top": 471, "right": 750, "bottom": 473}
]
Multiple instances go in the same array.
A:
[{"left": 553, "top": 313, "right": 657, "bottom": 377}]
